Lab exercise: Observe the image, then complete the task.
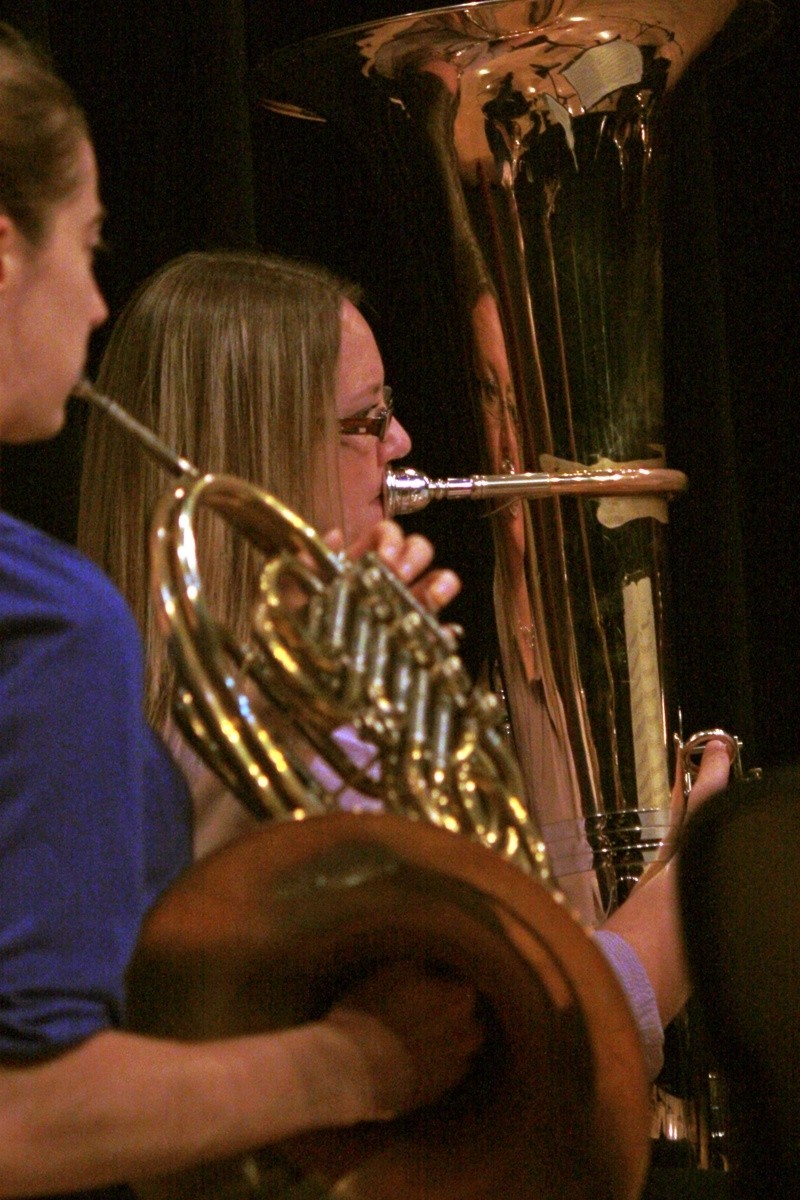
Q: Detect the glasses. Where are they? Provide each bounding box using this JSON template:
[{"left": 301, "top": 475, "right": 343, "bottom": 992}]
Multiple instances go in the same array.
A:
[{"left": 334, "top": 385, "right": 394, "bottom": 442}]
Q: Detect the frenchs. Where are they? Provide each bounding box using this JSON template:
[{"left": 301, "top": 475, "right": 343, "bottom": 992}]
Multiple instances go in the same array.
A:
[{"left": 66, "top": 376, "right": 649, "bottom": 1200}]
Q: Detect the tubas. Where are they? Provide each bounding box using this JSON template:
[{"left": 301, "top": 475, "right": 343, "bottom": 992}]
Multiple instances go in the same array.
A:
[{"left": 326, "top": 0, "right": 741, "bottom": 1200}]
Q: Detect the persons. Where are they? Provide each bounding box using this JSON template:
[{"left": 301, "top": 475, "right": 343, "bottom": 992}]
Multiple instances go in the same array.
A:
[
  {"left": 79, "top": 253, "right": 728, "bottom": 1083},
  {"left": 0, "top": 50, "right": 485, "bottom": 1200}
]
